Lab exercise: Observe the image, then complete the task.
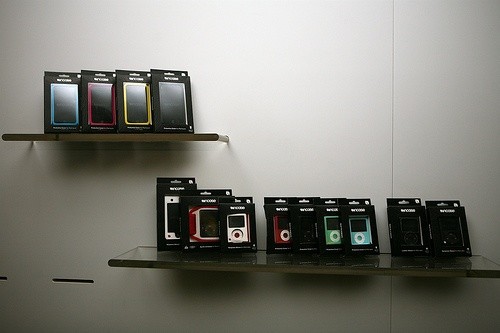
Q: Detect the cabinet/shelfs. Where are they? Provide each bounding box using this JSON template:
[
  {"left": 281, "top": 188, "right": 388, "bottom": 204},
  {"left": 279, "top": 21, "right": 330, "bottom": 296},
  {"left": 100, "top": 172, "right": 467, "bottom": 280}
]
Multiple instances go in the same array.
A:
[{"left": 2, "top": 133, "right": 500, "bottom": 280}]
